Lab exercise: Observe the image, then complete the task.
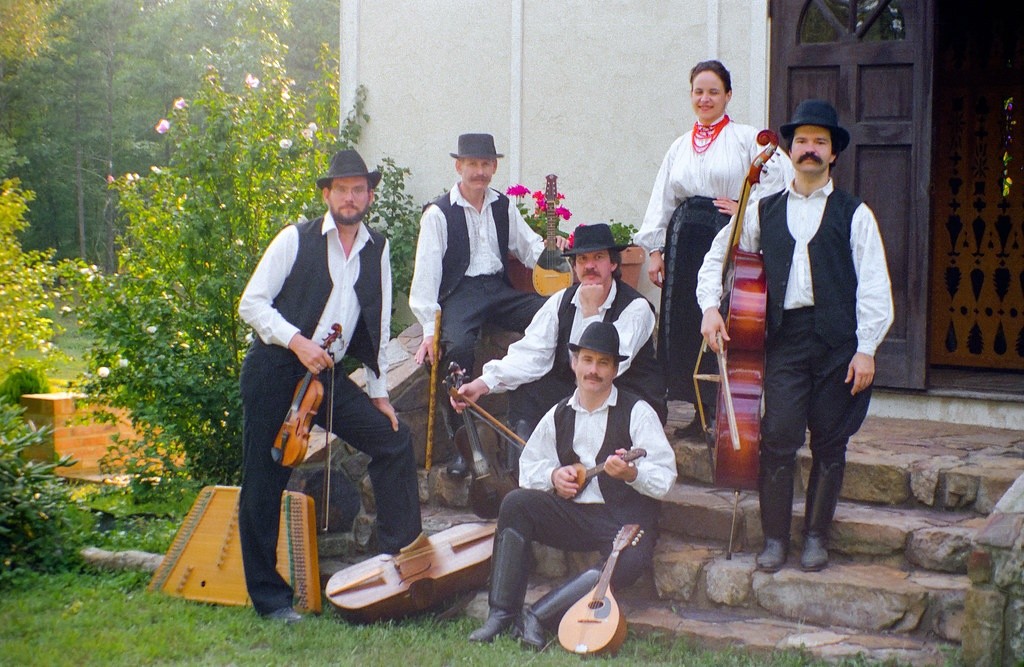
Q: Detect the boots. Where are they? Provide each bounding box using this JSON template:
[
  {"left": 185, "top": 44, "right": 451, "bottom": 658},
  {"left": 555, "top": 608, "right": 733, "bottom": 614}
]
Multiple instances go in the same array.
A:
[
  {"left": 756, "top": 460, "right": 795, "bottom": 574},
  {"left": 512, "top": 565, "right": 602, "bottom": 647},
  {"left": 798, "top": 459, "right": 843, "bottom": 573},
  {"left": 468, "top": 528, "right": 530, "bottom": 643}
]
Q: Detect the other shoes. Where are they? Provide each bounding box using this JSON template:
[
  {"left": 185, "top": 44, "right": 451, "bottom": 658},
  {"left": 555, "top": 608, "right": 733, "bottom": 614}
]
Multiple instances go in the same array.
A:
[
  {"left": 261, "top": 606, "right": 303, "bottom": 622},
  {"left": 674, "top": 400, "right": 716, "bottom": 445},
  {"left": 446, "top": 453, "right": 468, "bottom": 477}
]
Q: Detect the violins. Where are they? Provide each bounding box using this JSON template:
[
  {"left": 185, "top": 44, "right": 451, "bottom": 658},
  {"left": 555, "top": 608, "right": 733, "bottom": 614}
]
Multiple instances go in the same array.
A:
[
  {"left": 443, "top": 360, "right": 521, "bottom": 520},
  {"left": 271, "top": 319, "right": 342, "bottom": 466}
]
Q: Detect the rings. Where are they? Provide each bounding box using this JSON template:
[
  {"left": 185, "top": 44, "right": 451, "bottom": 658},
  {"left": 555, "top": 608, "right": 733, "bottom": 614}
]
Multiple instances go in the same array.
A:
[{"left": 316, "top": 364, "right": 320, "bottom": 368}]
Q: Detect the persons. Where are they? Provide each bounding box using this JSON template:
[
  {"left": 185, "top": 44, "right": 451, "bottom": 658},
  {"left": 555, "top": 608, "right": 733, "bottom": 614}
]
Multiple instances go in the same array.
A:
[
  {"left": 407, "top": 133, "right": 570, "bottom": 481},
  {"left": 468, "top": 322, "right": 678, "bottom": 660},
  {"left": 694, "top": 99, "right": 895, "bottom": 574},
  {"left": 632, "top": 60, "right": 797, "bottom": 437},
  {"left": 449, "top": 224, "right": 669, "bottom": 435},
  {"left": 235, "top": 150, "right": 422, "bottom": 625}
]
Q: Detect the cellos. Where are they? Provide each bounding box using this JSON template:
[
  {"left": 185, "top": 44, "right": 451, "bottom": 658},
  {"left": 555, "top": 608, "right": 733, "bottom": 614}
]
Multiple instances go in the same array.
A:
[
  {"left": 707, "top": 127, "right": 784, "bottom": 491},
  {"left": 321, "top": 519, "right": 494, "bottom": 616}
]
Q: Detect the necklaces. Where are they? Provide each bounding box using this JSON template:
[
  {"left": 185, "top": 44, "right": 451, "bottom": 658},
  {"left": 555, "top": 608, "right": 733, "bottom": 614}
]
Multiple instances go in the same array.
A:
[{"left": 692, "top": 115, "right": 730, "bottom": 155}]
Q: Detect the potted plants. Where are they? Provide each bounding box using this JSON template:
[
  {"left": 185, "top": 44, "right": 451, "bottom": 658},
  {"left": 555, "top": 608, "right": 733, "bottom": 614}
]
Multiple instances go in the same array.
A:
[{"left": 610, "top": 219, "right": 645, "bottom": 292}]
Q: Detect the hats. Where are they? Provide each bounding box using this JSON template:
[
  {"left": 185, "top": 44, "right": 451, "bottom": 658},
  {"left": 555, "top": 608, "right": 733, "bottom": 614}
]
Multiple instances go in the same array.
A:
[
  {"left": 779, "top": 99, "right": 850, "bottom": 152},
  {"left": 560, "top": 223, "right": 629, "bottom": 257},
  {"left": 567, "top": 321, "right": 630, "bottom": 362},
  {"left": 316, "top": 150, "right": 381, "bottom": 190},
  {"left": 448, "top": 133, "right": 505, "bottom": 159}
]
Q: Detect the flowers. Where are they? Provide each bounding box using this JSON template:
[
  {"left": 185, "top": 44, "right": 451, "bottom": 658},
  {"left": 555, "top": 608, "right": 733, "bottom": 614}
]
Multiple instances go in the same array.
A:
[{"left": 506, "top": 185, "right": 586, "bottom": 249}]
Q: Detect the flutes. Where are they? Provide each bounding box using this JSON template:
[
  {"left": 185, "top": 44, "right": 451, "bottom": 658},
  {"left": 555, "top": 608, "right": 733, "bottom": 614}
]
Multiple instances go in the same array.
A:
[{"left": 425, "top": 310, "right": 443, "bottom": 472}]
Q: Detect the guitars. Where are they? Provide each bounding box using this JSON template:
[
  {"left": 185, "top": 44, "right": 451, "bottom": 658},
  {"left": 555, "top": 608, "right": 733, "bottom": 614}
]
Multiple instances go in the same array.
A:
[
  {"left": 530, "top": 172, "right": 575, "bottom": 298},
  {"left": 559, "top": 517, "right": 646, "bottom": 658},
  {"left": 560, "top": 449, "right": 649, "bottom": 499}
]
